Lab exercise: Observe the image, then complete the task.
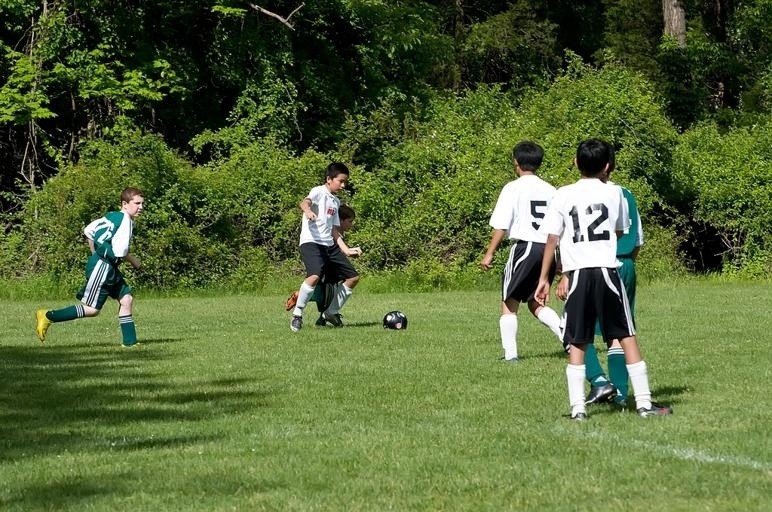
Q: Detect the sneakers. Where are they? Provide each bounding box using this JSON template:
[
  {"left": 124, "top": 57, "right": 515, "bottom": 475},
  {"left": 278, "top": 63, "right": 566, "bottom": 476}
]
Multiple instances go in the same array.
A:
[
  {"left": 285, "top": 291, "right": 298, "bottom": 311},
  {"left": 290, "top": 313, "right": 302, "bottom": 332},
  {"left": 121, "top": 342, "right": 141, "bottom": 349},
  {"left": 567, "top": 383, "right": 673, "bottom": 424},
  {"left": 36, "top": 310, "right": 52, "bottom": 342},
  {"left": 315, "top": 309, "right": 343, "bottom": 327}
]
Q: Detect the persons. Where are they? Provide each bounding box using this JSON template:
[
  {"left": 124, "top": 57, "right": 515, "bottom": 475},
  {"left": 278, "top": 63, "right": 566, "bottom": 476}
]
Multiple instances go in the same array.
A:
[
  {"left": 290, "top": 163, "right": 362, "bottom": 332},
  {"left": 534, "top": 140, "right": 673, "bottom": 422},
  {"left": 555, "top": 142, "right": 644, "bottom": 408},
  {"left": 35, "top": 187, "right": 145, "bottom": 346},
  {"left": 286, "top": 206, "right": 362, "bottom": 328},
  {"left": 480, "top": 141, "right": 571, "bottom": 360}
]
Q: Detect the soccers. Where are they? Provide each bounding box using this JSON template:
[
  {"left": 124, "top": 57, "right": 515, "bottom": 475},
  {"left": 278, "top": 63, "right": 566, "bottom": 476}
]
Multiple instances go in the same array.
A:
[{"left": 383, "top": 311, "right": 407, "bottom": 330}]
